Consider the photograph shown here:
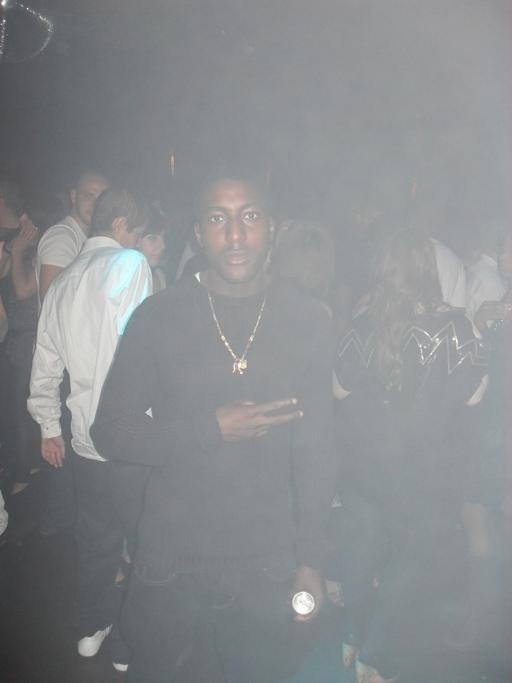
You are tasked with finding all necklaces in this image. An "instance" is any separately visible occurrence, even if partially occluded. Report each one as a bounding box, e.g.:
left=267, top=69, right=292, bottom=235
left=204, top=281, right=266, bottom=373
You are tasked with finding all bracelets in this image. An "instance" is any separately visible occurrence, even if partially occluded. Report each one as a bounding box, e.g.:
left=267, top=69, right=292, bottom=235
left=3, top=242, right=11, bottom=254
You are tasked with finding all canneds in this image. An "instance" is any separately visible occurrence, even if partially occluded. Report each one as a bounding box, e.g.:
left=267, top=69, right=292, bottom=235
left=288, top=590, right=315, bottom=615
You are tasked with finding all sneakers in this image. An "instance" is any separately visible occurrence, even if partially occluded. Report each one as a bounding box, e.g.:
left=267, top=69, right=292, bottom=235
left=75, top=620, right=113, bottom=659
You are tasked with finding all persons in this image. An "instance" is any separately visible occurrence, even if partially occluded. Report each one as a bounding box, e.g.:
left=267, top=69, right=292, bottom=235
left=0, top=164, right=194, bottom=682
left=276, top=165, right=512, bottom=682
left=89, top=167, right=342, bottom=683
